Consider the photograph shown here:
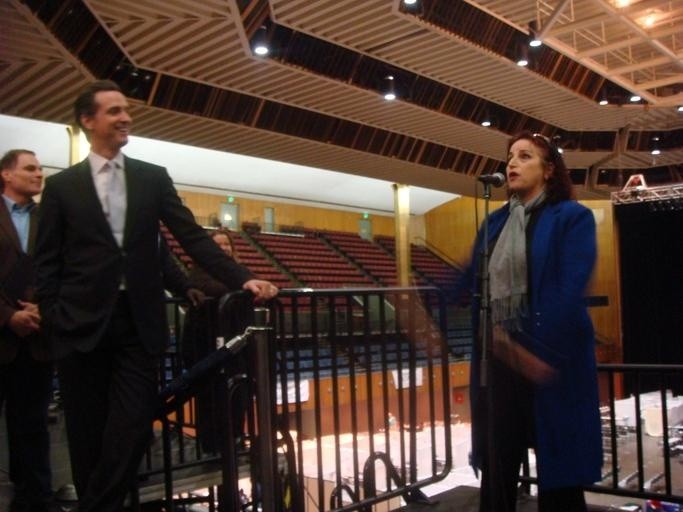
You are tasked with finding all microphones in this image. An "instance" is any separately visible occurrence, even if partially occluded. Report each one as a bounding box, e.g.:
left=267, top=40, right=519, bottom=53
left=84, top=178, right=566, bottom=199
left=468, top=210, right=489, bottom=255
left=478, top=172, right=506, bottom=188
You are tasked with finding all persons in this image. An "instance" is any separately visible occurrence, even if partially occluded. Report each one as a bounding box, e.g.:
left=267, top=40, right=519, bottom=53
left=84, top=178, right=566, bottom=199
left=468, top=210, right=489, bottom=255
left=447, top=131, right=607, bottom=511
left=0, top=149, right=60, bottom=510
left=33, top=78, right=279, bottom=510
left=158, top=243, right=205, bottom=310
left=175, top=229, right=247, bottom=472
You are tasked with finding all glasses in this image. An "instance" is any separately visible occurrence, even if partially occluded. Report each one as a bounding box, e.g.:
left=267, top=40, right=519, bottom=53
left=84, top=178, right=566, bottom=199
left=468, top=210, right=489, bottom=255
left=533, top=133, right=551, bottom=146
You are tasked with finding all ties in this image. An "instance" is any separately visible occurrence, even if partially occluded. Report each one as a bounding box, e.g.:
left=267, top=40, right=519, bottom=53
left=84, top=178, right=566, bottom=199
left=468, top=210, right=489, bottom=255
left=105, top=160, right=128, bottom=249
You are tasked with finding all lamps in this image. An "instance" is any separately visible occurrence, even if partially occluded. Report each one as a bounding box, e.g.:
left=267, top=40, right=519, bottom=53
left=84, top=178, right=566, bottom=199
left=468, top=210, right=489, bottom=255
left=382, top=74, right=396, bottom=102
left=403, top=0, right=681, bottom=158
left=253, top=23, right=271, bottom=57
